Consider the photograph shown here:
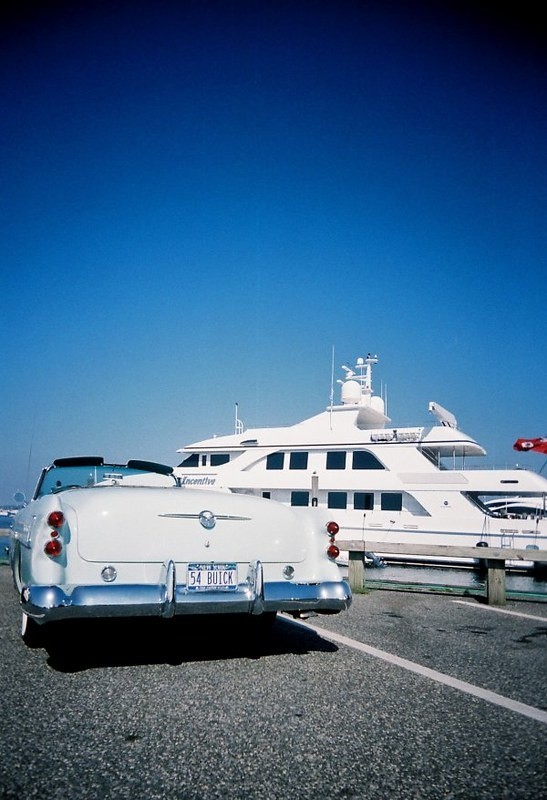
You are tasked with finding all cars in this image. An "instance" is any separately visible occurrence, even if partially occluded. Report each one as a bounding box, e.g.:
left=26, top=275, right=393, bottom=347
left=9, top=454, right=352, bottom=648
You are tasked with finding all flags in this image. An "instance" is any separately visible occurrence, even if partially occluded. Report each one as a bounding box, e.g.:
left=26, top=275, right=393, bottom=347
left=513, top=435, right=547, bottom=454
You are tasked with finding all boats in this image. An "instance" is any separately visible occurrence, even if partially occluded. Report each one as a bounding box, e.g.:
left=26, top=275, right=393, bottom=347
left=169, top=344, right=547, bottom=571
